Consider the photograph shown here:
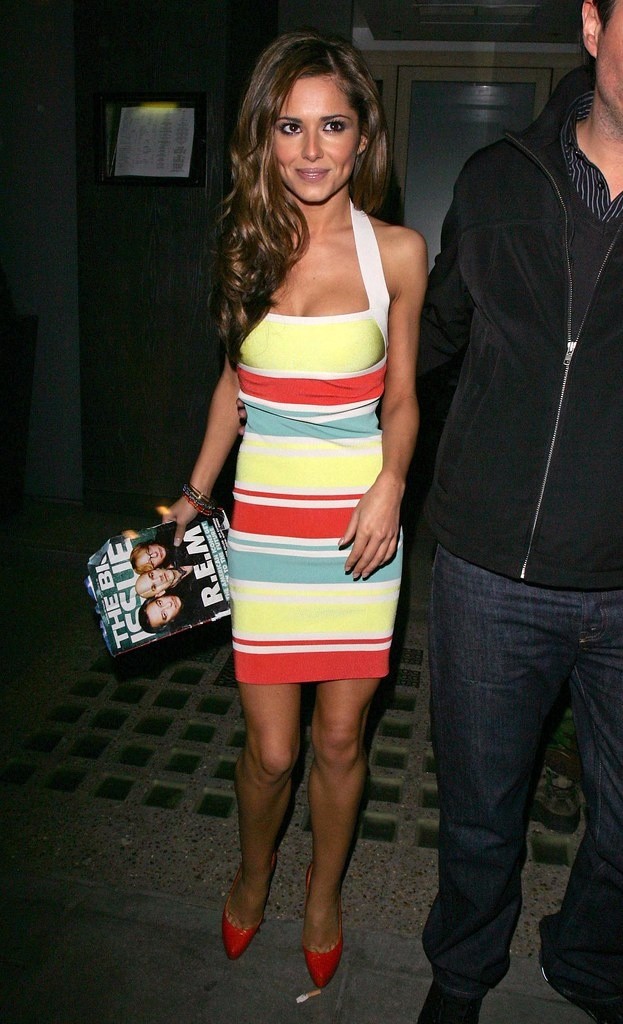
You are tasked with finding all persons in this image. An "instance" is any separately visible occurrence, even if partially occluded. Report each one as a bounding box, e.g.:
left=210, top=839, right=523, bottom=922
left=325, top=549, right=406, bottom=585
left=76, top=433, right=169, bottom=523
left=163, top=29, right=427, bottom=989
left=235, top=0, right=623, bottom=1023
left=130, top=522, right=229, bottom=633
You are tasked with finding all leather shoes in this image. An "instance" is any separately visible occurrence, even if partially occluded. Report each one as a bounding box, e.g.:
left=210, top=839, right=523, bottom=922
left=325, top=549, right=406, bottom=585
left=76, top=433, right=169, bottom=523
left=305, top=864, right=342, bottom=988
left=222, top=852, right=277, bottom=960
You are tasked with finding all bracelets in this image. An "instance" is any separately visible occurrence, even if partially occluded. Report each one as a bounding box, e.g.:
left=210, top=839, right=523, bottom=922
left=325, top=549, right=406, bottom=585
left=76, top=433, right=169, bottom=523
left=181, top=481, right=218, bottom=516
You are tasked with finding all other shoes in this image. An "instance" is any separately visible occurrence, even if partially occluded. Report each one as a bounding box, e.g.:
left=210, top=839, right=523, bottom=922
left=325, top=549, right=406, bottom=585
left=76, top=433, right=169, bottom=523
left=541, top=963, right=623, bottom=1024
left=417, top=980, right=484, bottom=1024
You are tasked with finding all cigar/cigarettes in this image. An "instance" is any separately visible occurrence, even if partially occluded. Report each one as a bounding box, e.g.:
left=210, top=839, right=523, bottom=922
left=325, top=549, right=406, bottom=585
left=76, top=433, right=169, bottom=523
left=296, top=989, right=321, bottom=1003
